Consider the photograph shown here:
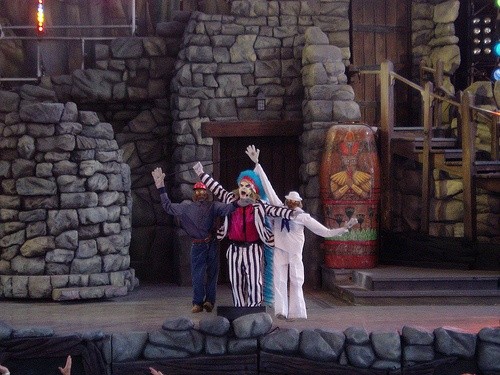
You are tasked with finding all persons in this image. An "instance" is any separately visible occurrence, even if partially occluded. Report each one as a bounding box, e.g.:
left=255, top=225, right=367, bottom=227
left=194, top=161, right=303, bottom=307
left=151, top=167, right=256, bottom=314
left=59, top=355, right=71, bottom=375
left=246, top=145, right=359, bottom=320
left=149, top=367, right=163, bottom=375
left=0, top=365, right=11, bottom=375
